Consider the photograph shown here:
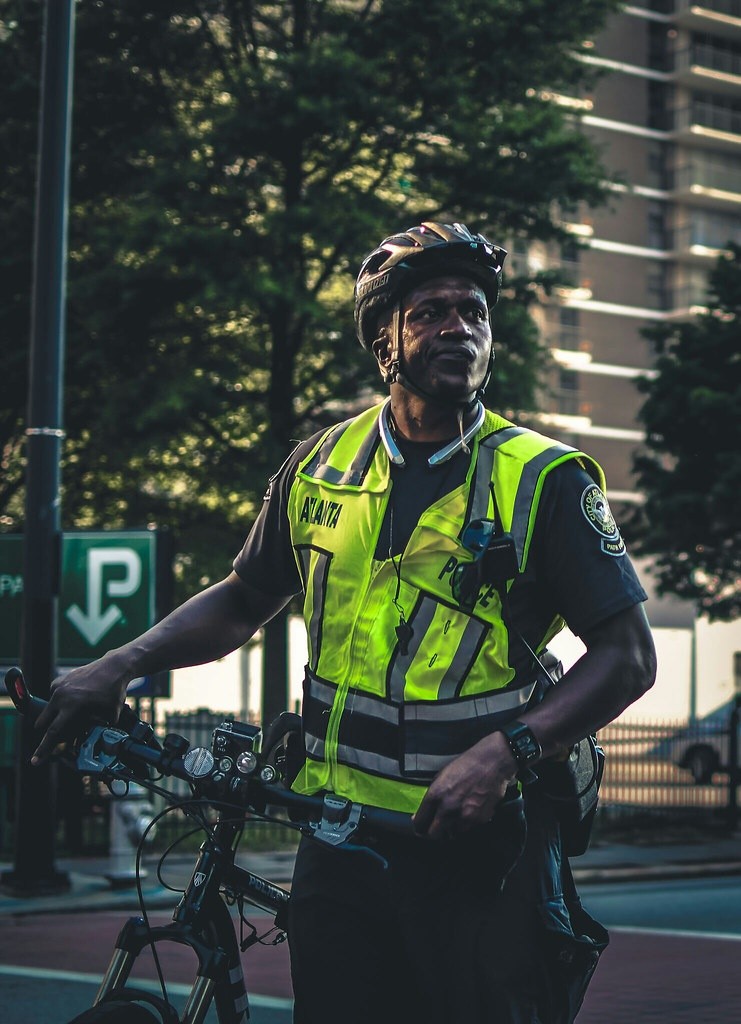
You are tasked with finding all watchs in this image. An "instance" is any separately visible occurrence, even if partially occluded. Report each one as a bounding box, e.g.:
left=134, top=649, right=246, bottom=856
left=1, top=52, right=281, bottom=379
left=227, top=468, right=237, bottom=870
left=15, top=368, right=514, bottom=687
left=500, top=723, right=544, bottom=786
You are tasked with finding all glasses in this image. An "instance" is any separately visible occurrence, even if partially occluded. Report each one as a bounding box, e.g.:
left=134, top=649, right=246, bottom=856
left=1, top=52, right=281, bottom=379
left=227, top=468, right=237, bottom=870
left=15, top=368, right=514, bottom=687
left=451, top=519, right=497, bottom=610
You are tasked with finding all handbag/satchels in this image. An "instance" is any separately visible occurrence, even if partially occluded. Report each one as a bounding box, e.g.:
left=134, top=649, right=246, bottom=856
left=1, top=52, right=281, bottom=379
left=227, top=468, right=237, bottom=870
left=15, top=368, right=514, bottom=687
left=531, top=809, right=610, bottom=1024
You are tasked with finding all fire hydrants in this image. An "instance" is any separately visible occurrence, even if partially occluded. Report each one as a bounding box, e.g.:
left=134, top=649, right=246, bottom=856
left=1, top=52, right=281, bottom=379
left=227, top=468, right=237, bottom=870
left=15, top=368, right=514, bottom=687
left=97, top=775, right=160, bottom=889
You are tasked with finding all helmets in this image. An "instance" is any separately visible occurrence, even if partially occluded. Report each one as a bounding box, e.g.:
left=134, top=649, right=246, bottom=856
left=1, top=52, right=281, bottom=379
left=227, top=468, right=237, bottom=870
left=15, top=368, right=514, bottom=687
left=352, top=221, right=508, bottom=352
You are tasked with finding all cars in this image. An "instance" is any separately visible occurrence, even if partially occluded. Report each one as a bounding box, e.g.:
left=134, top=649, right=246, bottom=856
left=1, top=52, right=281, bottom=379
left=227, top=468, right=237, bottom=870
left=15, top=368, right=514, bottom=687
left=668, top=732, right=732, bottom=784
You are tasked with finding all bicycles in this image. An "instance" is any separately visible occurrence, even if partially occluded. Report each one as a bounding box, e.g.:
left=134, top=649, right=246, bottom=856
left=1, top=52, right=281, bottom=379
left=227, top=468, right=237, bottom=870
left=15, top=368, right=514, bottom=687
left=5, top=664, right=530, bottom=1024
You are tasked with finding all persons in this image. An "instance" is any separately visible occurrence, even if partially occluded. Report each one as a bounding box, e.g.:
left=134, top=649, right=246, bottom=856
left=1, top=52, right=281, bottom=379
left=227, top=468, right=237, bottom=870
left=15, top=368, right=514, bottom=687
left=711, top=651, right=741, bottom=821
left=27, top=218, right=660, bottom=1023
